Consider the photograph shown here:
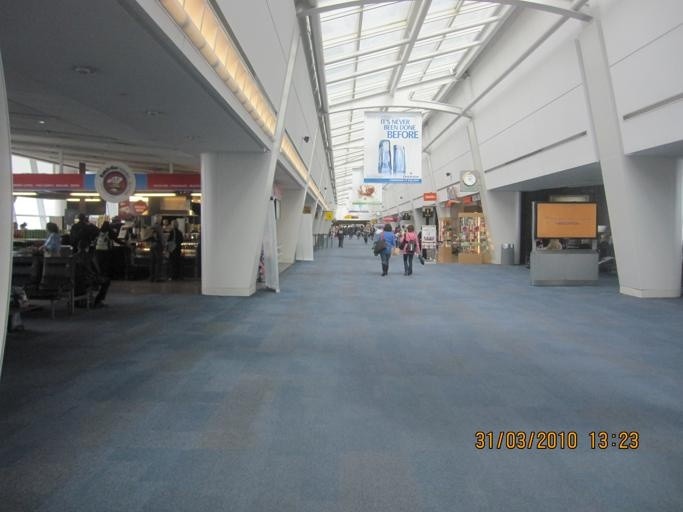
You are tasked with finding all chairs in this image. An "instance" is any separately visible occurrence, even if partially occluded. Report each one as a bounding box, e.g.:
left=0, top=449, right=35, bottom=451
left=9, top=254, right=100, bottom=321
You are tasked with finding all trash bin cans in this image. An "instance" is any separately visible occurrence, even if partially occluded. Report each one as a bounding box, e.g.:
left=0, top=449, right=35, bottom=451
left=501, top=243, right=515, bottom=266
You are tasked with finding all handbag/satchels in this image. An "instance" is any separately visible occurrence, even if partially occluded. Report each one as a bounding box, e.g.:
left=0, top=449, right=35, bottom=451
left=374, top=239, right=386, bottom=256
left=399, top=239, right=406, bottom=250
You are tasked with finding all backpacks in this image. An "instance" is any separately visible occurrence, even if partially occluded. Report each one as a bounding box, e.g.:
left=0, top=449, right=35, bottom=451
left=407, top=240, right=416, bottom=252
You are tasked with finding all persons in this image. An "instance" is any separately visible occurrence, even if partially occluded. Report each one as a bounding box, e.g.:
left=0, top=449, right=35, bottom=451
left=336, top=223, right=427, bottom=276
left=14, top=213, right=184, bottom=309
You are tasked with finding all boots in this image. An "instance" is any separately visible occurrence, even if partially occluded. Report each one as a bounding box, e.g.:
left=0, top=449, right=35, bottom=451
left=381, top=263, right=388, bottom=276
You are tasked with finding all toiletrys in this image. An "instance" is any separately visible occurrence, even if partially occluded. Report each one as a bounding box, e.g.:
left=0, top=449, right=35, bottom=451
left=379, top=139, right=392, bottom=174
left=393, top=144, right=406, bottom=174
